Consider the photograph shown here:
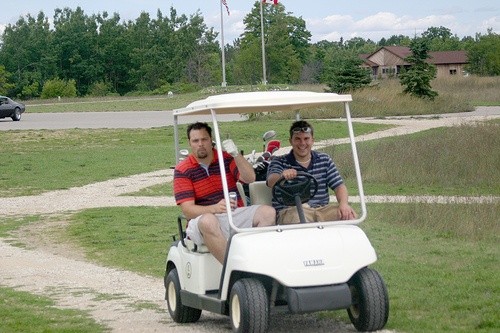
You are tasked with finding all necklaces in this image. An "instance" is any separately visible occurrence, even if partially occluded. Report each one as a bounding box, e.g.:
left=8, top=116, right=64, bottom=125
left=297, top=157, right=312, bottom=164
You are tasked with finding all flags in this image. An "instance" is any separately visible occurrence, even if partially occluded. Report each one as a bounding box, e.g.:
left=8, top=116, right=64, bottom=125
left=222, top=0, right=230, bottom=15
left=262, top=0, right=278, bottom=4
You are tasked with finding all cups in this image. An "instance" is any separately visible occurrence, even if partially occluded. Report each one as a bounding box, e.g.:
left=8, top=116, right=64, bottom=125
left=229, top=192, right=237, bottom=212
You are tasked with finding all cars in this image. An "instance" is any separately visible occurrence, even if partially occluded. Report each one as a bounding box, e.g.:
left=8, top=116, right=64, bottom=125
left=0, top=96, right=26, bottom=121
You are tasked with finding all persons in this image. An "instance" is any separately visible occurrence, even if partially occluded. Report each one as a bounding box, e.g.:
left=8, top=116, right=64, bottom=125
left=267, top=120, right=356, bottom=225
left=173, top=123, right=276, bottom=267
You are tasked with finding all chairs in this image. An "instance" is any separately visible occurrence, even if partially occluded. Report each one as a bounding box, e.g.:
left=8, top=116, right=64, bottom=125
left=249, top=181, right=274, bottom=207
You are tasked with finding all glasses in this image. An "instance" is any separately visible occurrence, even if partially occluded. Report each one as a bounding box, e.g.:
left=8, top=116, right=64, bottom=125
left=291, top=127, right=313, bottom=133
